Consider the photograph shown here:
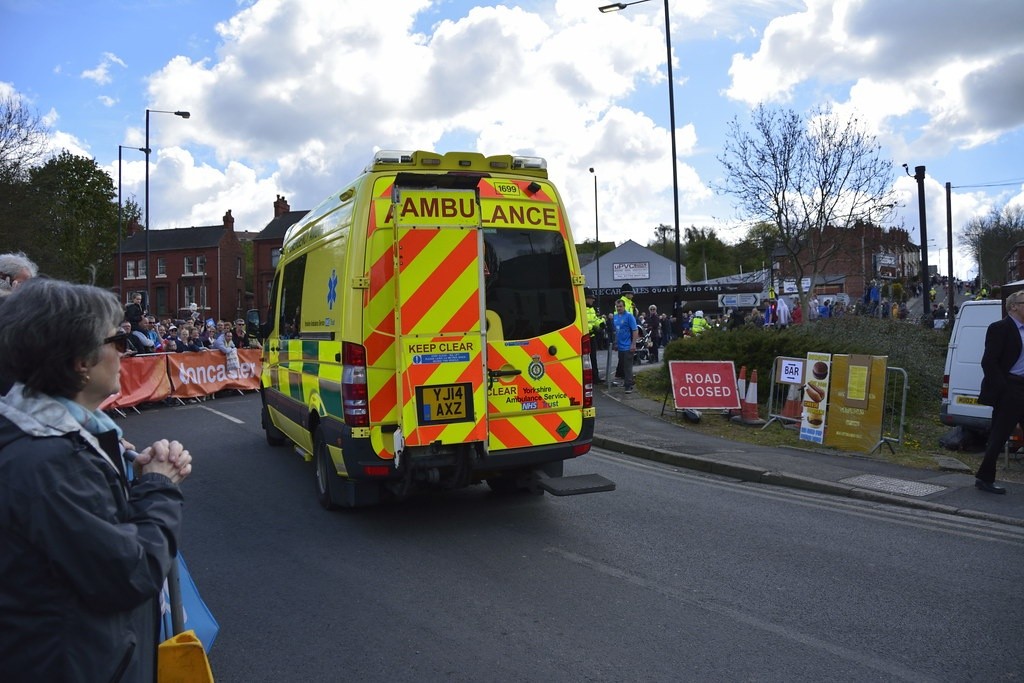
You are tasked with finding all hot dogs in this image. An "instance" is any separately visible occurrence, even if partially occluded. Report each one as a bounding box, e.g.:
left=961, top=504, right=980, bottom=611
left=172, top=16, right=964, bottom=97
left=804, top=382, right=825, bottom=402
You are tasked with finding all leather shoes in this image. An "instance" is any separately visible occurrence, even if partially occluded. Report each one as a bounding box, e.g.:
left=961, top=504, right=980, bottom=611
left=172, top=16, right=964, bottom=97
left=975, top=478, right=1006, bottom=494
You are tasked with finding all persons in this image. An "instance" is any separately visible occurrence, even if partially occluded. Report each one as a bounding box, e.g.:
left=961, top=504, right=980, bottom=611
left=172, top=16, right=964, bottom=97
left=0, top=251, right=266, bottom=683
left=579, top=271, right=1023, bottom=493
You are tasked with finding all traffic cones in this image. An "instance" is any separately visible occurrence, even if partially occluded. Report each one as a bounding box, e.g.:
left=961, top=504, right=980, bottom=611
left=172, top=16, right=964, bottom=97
left=725, top=366, right=748, bottom=419
left=738, top=368, right=766, bottom=426
left=780, top=380, right=804, bottom=432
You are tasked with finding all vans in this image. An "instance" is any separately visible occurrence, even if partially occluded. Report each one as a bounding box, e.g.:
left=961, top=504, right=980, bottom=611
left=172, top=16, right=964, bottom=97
left=939, top=299, right=1024, bottom=453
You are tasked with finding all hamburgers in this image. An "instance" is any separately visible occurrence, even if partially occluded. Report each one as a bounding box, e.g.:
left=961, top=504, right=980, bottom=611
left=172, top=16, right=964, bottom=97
left=813, top=362, right=828, bottom=380
left=807, top=411, right=823, bottom=427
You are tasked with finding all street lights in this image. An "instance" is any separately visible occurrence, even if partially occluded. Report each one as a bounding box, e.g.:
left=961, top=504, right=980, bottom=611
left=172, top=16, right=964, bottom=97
left=115, top=143, right=153, bottom=300
left=599, top=0, right=690, bottom=340
left=589, top=167, right=601, bottom=314
left=145, top=106, right=193, bottom=333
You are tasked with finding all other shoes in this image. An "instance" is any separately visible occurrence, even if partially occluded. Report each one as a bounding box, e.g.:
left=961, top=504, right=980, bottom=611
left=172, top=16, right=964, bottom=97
left=647, top=359, right=659, bottom=364
left=593, top=378, right=637, bottom=394
left=147, top=394, right=211, bottom=406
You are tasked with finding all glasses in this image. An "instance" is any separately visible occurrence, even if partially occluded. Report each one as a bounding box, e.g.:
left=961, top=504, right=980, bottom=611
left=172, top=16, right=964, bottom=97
left=149, top=322, right=155, bottom=324
left=650, top=308, right=655, bottom=310
left=104, top=331, right=130, bottom=353
left=224, top=328, right=229, bottom=329
left=209, top=331, right=214, bottom=333
left=238, top=324, right=244, bottom=326
left=187, top=322, right=194, bottom=324
left=1015, top=301, right=1024, bottom=304
left=169, top=329, right=177, bottom=332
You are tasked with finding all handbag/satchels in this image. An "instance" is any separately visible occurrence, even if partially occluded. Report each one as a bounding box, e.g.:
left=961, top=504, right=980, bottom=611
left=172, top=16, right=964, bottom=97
left=126, top=454, right=221, bottom=657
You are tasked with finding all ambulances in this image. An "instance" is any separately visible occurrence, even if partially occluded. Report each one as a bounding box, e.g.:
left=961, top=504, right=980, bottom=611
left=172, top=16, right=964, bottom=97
left=246, top=153, right=616, bottom=513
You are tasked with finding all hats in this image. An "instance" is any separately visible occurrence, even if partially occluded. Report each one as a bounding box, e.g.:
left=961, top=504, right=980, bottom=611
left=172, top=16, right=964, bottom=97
left=169, top=325, right=177, bottom=329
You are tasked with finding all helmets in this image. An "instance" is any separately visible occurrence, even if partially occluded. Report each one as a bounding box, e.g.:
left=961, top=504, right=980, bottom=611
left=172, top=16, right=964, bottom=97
left=620, top=284, right=634, bottom=293
left=695, top=310, right=703, bottom=317
left=584, top=287, right=597, bottom=299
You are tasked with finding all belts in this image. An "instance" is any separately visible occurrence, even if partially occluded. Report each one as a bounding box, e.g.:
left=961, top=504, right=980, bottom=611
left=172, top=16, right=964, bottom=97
left=1008, top=375, right=1024, bottom=383
